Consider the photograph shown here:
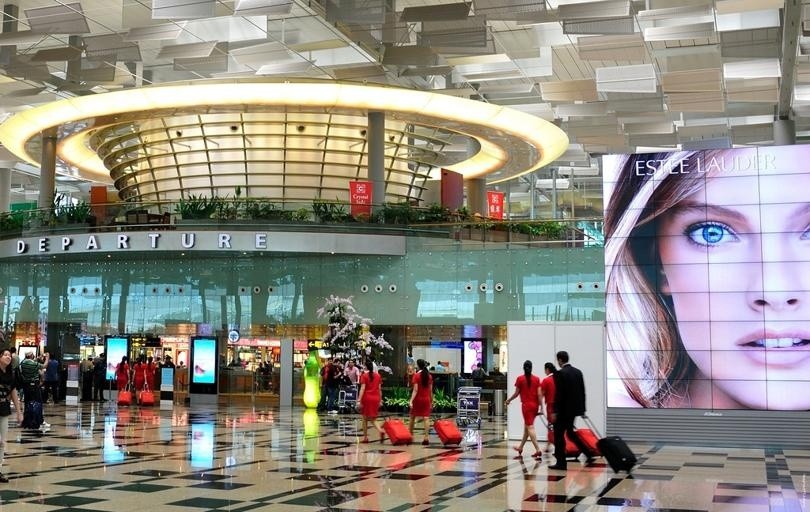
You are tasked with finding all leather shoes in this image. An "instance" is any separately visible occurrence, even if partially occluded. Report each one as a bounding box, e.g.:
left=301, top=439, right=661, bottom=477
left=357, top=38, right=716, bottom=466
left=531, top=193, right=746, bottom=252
left=547, top=462, right=570, bottom=470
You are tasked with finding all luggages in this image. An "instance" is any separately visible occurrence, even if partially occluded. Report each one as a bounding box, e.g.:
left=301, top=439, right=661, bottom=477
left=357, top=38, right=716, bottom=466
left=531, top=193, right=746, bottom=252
left=384, top=418, right=413, bottom=446
left=433, top=418, right=463, bottom=446
left=548, top=428, right=601, bottom=458
left=117, top=382, right=133, bottom=405
left=597, top=435, right=637, bottom=475
left=25, top=400, right=42, bottom=429
left=140, top=384, right=155, bottom=406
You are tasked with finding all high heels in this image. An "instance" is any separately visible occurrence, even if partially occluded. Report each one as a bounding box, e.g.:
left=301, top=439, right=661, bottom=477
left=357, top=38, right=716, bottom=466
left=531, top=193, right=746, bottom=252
left=357, top=429, right=430, bottom=446
left=512, top=441, right=553, bottom=458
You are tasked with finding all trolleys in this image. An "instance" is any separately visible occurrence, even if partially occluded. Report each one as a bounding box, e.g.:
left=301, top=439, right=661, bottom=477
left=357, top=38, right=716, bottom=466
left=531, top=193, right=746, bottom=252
left=453, top=386, right=483, bottom=431
left=339, top=384, right=360, bottom=414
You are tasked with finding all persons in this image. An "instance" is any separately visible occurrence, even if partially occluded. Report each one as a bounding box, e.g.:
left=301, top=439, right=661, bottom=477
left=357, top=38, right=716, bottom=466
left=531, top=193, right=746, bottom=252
left=489, top=366, right=504, bottom=376
left=0, top=348, right=24, bottom=483
left=356, top=360, right=385, bottom=444
left=426, top=361, right=445, bottom=372
left=408, top=358, right=434, bottom=445
left=10, top=346, right=175, bottom=405
left=20, top=351, right=51, bottom=429
left=318, top=357, right=360, bottom=414
left=604, top=144, right=810, bottom=410
left=471, top=363, right=487, bottom=387
left=228, top=357, right=272, bottom=393
left=541, top=362, right=558, bottom=453
left=548, top=350, right=596, bottom=470
left=505, top=360, right=542, bottom=458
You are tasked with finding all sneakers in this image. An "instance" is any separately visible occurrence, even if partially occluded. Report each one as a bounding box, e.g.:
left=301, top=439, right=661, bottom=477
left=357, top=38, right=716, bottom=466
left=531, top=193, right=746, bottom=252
left=0, top=472, right=9, bottom=483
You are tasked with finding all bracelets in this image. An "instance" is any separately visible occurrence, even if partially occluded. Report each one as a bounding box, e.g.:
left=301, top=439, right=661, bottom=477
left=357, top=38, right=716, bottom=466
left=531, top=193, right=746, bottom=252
left=357, top=401, right=360, bottom=402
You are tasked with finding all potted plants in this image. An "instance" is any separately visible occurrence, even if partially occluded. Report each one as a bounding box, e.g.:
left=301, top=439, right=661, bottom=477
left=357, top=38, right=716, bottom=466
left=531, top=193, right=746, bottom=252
left=381, top=385, right=459, bottom=413
